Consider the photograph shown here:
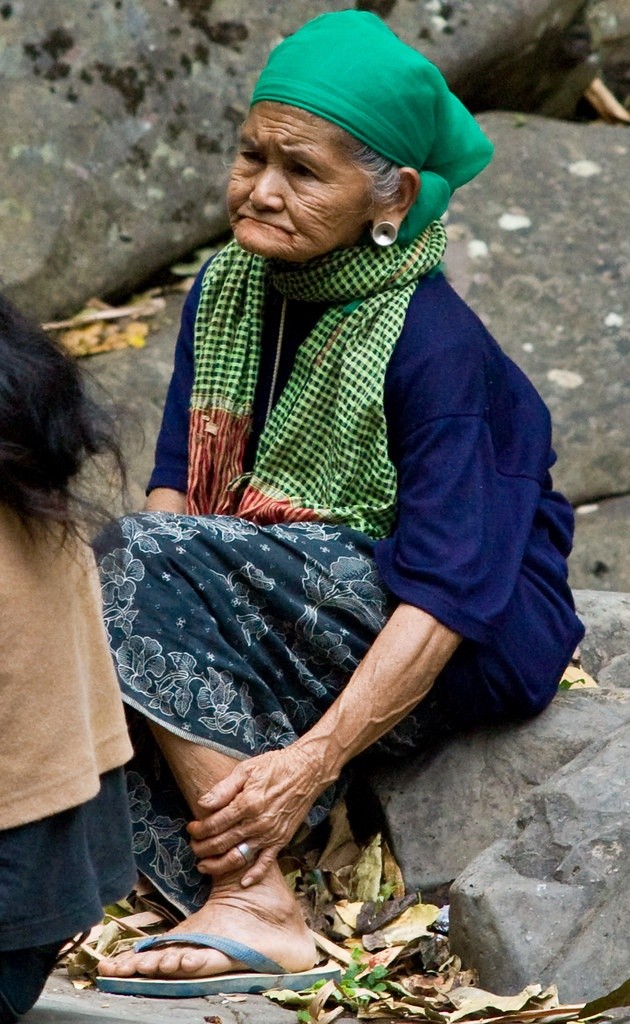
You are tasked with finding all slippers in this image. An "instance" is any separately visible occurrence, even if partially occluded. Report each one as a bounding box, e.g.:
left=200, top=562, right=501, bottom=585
left=94, top=933, right=340, bottom=997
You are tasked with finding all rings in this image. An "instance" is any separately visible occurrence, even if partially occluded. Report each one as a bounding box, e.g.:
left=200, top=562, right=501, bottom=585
left=237, top=842, right=254, bottom=863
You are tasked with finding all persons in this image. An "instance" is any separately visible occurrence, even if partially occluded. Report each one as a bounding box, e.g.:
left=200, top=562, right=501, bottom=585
left=0, top=297, right=139, bottom=1024
left=66, top=9, right=587, bottom=997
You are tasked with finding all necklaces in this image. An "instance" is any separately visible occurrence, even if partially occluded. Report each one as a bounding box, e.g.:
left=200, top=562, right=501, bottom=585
left=264, top=296, right=287, bottom=424
